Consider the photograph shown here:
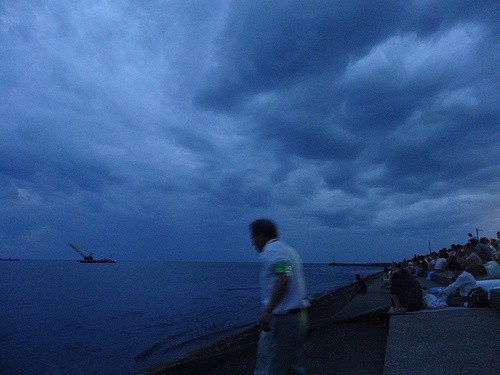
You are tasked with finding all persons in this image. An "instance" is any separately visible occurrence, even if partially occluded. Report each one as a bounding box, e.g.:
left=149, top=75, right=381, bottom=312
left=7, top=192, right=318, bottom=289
left=349, top=274, right=367, bottom=300
left=250, top=219, right=309, bottom=375
left=381, top=231, right=500, bottom=313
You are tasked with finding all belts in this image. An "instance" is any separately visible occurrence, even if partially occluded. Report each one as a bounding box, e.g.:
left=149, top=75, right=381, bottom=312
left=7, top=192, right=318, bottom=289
left=288, top=309, right=302, bottom=315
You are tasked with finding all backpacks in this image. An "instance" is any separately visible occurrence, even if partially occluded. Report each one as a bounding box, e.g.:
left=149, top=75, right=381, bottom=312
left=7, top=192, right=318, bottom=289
left=466, top=287, right=487, bottom=308
